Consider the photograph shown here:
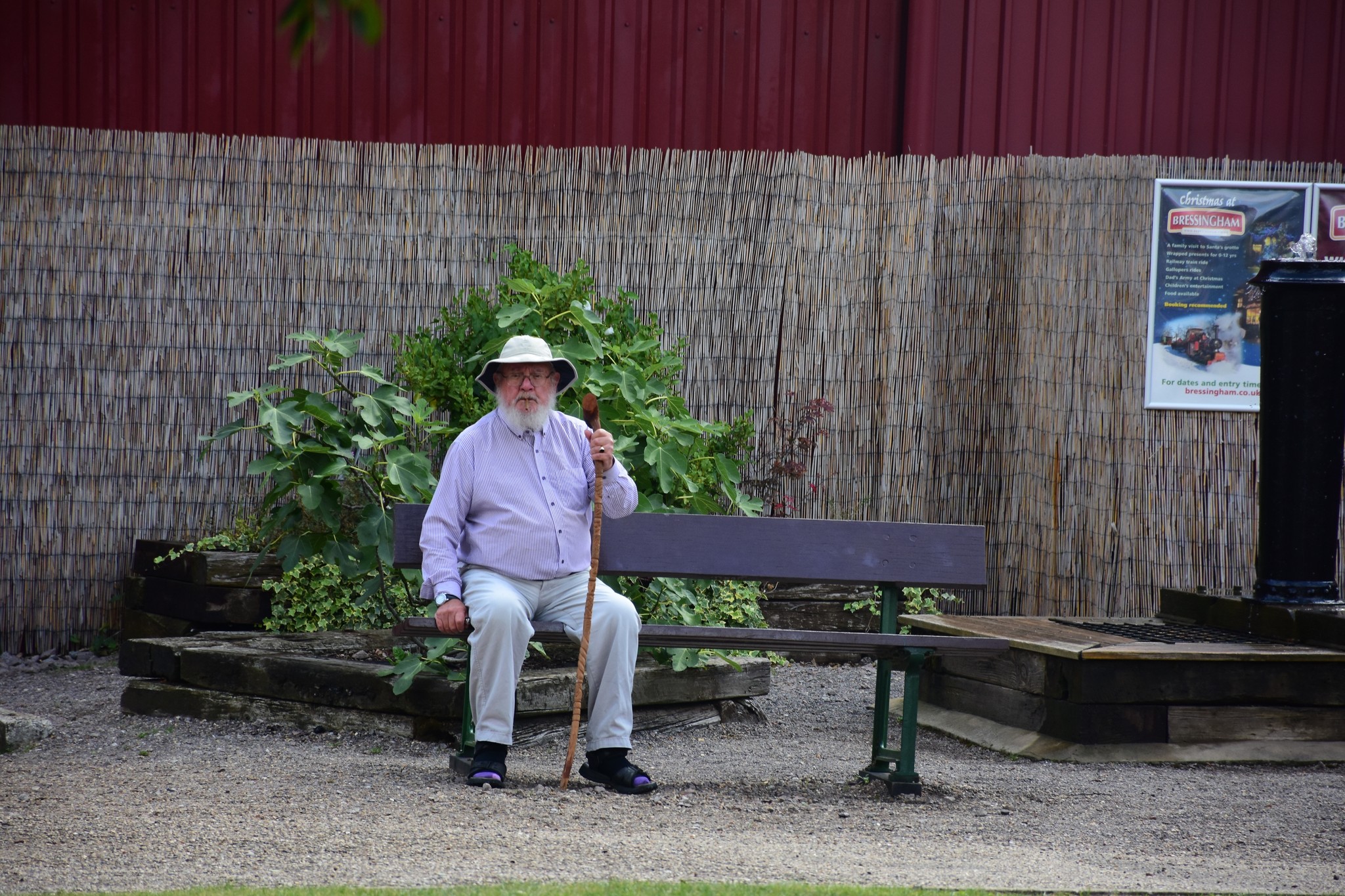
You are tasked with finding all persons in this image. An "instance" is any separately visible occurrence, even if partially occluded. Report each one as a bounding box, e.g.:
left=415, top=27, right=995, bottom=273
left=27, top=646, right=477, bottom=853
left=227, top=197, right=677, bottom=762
left=419, top=335, right=659, bottom=795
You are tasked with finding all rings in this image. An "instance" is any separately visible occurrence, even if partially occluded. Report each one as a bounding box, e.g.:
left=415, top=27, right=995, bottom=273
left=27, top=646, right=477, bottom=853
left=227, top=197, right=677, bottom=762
left=599, top=446, right=605, bottom=453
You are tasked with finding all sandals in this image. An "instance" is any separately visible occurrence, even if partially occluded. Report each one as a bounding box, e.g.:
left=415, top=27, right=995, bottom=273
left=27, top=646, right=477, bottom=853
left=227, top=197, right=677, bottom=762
left=578, top=747, right=658, bottom=793
left=465, top=740, right=508, bottom=788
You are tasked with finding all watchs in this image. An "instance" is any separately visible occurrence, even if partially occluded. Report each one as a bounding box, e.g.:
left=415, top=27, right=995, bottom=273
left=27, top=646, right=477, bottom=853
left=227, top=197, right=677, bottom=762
left=435, top=592, right=460, bottom=608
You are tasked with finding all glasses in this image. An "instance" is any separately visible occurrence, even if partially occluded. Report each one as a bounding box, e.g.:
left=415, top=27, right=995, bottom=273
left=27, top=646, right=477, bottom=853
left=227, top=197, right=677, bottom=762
left=497, top=369, right=553, bottom=387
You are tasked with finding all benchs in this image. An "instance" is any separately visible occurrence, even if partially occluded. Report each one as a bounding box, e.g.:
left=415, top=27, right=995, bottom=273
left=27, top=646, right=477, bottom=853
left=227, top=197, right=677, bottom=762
left=393, top=501, right=1010, bottom=798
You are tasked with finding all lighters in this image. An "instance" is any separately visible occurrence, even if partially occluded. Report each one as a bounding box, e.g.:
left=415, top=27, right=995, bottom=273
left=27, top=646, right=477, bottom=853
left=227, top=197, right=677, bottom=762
left=464, top=617, right=470, bottom=625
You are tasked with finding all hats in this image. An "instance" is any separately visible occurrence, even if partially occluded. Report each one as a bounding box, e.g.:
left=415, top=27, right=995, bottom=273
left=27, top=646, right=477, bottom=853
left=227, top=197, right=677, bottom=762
left=475, top=334, right=578, bottom=398
